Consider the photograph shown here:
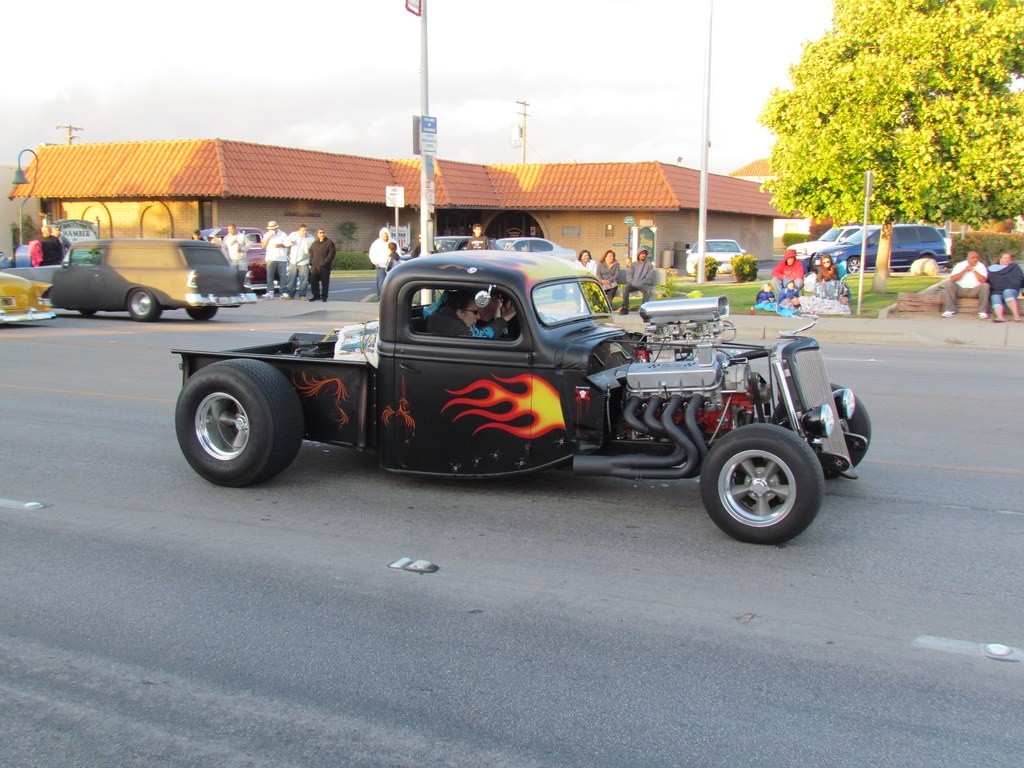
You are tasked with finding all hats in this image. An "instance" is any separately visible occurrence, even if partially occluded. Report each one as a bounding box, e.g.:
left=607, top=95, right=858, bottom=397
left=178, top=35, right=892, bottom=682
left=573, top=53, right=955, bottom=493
left=474, top=287, right=502, bottom=312
left=267, top=221, right=279, bottom=229
left=194, top=229, right=200, bottom=235
left=441, top=290, right=475, bottom=310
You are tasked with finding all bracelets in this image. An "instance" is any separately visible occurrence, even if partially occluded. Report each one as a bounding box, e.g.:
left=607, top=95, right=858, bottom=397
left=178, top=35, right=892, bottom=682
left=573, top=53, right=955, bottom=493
left=972, top=269, right=976, bottom=273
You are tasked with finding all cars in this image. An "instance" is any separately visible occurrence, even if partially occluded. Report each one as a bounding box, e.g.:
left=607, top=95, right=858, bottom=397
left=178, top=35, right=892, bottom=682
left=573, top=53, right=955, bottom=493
left=812, top=224, right=949, bottom=278
left=3, top=238, right=262, bottom=319
left=787, top=226, right=862, bottom=274
left=390, top=237, right=502, bottom=280
left=496, top=237, right=578, bottom=268
left=196, top=227, right=311, bottom=293
left=685, top=239, right=747, bottom=278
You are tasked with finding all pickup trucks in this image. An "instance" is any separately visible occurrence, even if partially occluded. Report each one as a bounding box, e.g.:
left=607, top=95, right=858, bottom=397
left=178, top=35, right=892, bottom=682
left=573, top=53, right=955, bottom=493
left=169, top=251, right=873, bottom=547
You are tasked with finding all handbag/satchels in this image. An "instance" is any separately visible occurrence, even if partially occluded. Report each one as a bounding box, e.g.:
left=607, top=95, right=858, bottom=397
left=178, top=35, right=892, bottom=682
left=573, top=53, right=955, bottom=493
left=603, top=281, right=618, bottom=291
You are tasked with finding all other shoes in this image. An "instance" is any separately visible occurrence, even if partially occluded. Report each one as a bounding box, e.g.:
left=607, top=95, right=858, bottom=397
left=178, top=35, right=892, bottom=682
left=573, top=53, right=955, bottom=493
left=619, top=308, right=628, bottom=315
left=1015, top=318, right=1021, bottom=321
left=323, top=297, right=326, bottom=302
left=283, top=295, right=293, bottom=300
left=976, top=312, right=989, bottom=319
left=280, top=292, right=289, bottom=298
left=299, top=295, right=306, bottom=301
left=993, top=317, right=1006, bottom=321
left=309, top=296, right=320, bottom=301
left=261, top=291, right=274, bottom=298
left=941, top=311, right=955, bottom=318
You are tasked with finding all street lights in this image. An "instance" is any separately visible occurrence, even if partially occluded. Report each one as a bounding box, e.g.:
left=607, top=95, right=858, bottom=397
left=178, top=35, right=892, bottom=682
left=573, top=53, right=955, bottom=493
left=12, top=146, right=38, bottom=247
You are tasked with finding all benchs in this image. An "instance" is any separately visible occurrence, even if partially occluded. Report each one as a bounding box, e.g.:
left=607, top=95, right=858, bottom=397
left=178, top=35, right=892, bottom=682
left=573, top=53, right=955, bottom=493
left=410, top=306, right=427, bottom=334
left=897, top=292, right=1024, bottom=316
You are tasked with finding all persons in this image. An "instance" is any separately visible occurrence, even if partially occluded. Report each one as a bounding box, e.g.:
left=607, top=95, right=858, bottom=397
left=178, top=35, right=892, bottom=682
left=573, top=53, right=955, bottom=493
left=426, top=289, right=517, bottom=340
left=368, top=227, right=397, bottom=301
left=411, top=234, right=438, bottom=259
left=575, top=249, right=597, bottom=276
left=618, top=247, right=655, bottom=315
left=987, top=251, right=1024, bottom=322
left=595, top=250, right=620, bottom=307
left=465, top=224, right=491, bottom=250
left=384, top=242, right=401, bottom=276
left=192, top=219, right=336, bottom=302
left=754, top=249, right=840, bottom=310
left=31, top=223, right=71, bottom=267
left=940, top=250, right=990, bottom=318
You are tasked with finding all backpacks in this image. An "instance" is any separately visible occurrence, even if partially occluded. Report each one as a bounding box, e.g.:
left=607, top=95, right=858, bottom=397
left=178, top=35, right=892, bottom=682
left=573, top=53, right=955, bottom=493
left=387, top=255, right=402, bottom=267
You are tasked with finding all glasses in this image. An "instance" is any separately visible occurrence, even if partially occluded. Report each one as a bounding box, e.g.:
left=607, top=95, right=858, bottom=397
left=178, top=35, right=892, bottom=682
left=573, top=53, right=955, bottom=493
left=465, top=310, right=480, bottom=315
left=317, top=231, right=325, bottom=234
left=823, top=261, right=829, bottom=264
